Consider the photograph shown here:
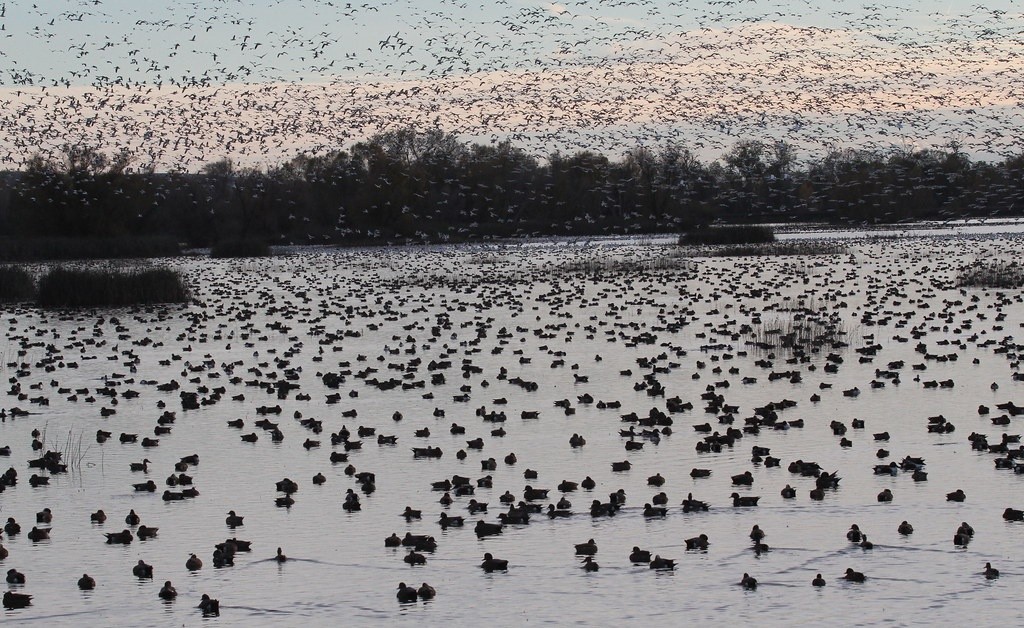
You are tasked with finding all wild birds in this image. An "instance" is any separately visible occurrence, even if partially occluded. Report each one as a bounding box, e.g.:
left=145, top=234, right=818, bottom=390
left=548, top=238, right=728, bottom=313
left=0, top=2, right=1024, bottom=296
left=0, top=295, right=1024, bottom=627
